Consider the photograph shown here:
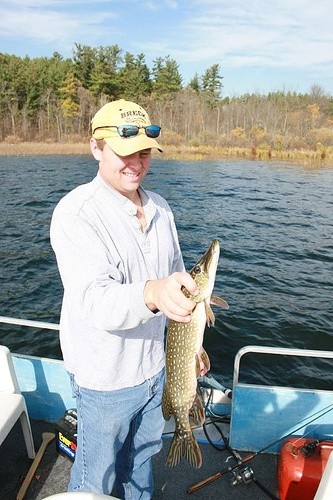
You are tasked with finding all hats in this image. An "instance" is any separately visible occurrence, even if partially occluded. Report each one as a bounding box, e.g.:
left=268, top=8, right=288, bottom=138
left=92, top=98, right=163, bottom=157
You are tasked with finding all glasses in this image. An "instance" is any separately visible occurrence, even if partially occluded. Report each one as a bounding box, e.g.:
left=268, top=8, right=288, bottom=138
left=93, top=125, right=161, bottom=138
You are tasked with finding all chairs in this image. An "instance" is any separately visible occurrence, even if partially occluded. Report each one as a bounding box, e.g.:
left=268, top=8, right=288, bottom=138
left=0, top=344, right=36, bottom=460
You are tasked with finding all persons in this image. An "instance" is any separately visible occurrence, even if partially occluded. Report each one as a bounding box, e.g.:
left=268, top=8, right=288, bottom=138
left=49, top=99, right=199, bottom=500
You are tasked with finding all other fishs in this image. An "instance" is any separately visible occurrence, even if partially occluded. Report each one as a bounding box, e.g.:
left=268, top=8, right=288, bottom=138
left=161, top=238, right=230, bottom=471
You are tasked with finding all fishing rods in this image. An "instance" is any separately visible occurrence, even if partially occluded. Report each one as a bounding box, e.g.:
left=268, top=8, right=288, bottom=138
left=189, top=403, right=333, bottom=492
